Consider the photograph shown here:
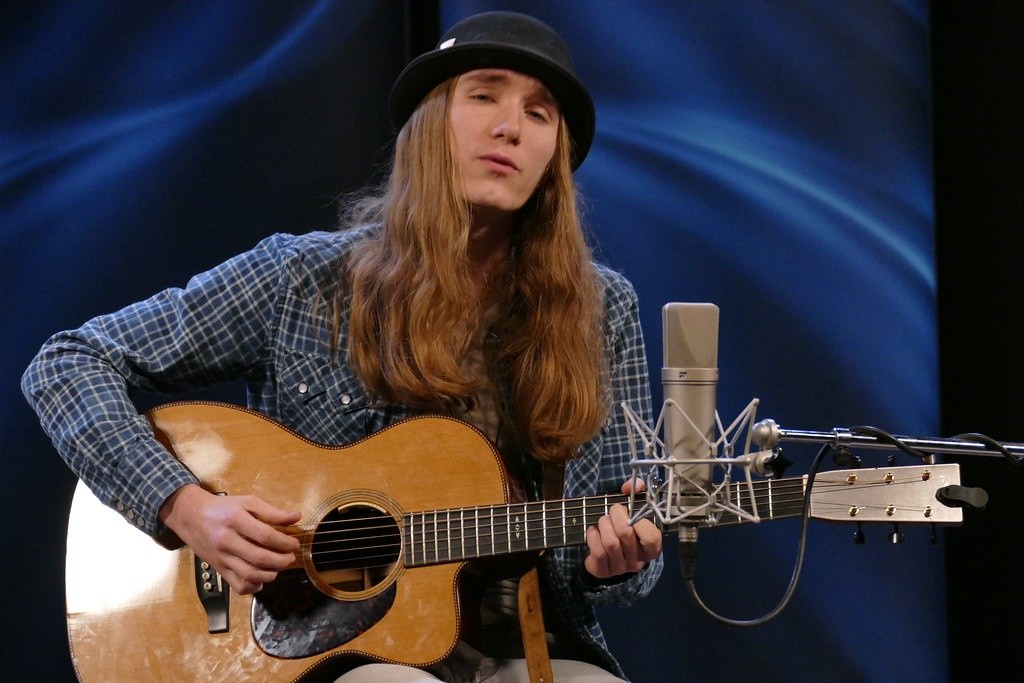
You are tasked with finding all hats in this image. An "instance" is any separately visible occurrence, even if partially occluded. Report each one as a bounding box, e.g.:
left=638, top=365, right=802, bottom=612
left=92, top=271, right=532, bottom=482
left=389, top=10, right=596, bottom=175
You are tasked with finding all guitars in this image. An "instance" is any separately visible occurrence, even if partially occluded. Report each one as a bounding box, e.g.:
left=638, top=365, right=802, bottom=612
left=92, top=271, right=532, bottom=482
left=64, top=400, right=989, bottom=682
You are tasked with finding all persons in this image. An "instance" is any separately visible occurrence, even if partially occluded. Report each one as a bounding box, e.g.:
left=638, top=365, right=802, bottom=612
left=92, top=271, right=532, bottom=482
left=20, top=11, right=665, bottom=683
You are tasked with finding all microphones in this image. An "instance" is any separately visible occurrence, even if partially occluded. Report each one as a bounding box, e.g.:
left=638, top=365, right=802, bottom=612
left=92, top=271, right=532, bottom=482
left=661, top=303, right=719, bottom=518
